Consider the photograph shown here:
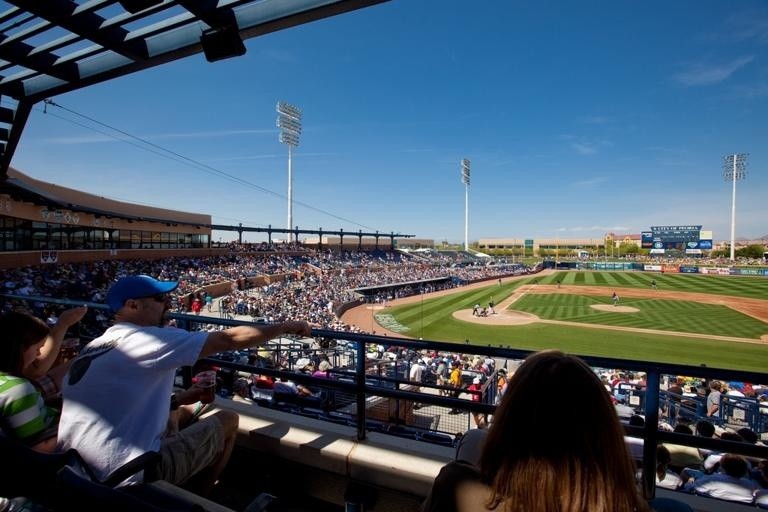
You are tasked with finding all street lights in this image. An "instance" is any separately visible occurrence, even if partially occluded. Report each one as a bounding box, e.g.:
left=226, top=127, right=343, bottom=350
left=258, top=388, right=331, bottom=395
left=460, top=157, right=472, bottom=251
left=274, top=99, right=304, bottom=245
left=720, top=151, right=750, bottom=261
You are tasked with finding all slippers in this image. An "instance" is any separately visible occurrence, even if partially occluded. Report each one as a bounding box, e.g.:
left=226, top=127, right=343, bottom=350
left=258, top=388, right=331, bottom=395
left=187, top=401, right=211, bottom=423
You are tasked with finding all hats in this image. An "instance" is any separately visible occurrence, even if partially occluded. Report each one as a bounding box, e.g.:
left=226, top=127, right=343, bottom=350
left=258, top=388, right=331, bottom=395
left=294, top=358, right=310, bottom=370
left=234, top=377, right=252, bottom=390
left=106, top=274, right=179, bottom=314
left=318, top=361, right=329, bottom=371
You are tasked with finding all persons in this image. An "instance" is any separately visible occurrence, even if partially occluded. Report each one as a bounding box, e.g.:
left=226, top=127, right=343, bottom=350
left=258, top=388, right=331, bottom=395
left=533, top=254, right=767, bottom=269
left=612, top=292, right=619, bottom=305
left=556, top=279, right=560, bottom=289
left=471, top=301, right=496, bottom=318
left=421, top=347, right=649, bottom=510
left=533, top=277, right=539, bottom=285
left=651, top=279, right=657, bottom=289
left=346, top=322, right=508, bottom=429
left=346, top=244, right=532, bottom=308
left=1, top=240, right=346, bottom=509
left=660, top=269, right=665, bottom=275
left=597, top=367, right=768, bottom=504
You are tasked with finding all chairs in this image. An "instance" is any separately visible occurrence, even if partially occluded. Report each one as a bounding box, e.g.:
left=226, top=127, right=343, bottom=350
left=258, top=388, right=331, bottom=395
left=86, top=452, right=366, bottom=512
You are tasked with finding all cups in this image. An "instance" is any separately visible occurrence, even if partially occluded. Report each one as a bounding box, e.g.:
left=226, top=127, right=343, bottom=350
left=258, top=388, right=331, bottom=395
left=194, top=370, right=216, bottom=404
